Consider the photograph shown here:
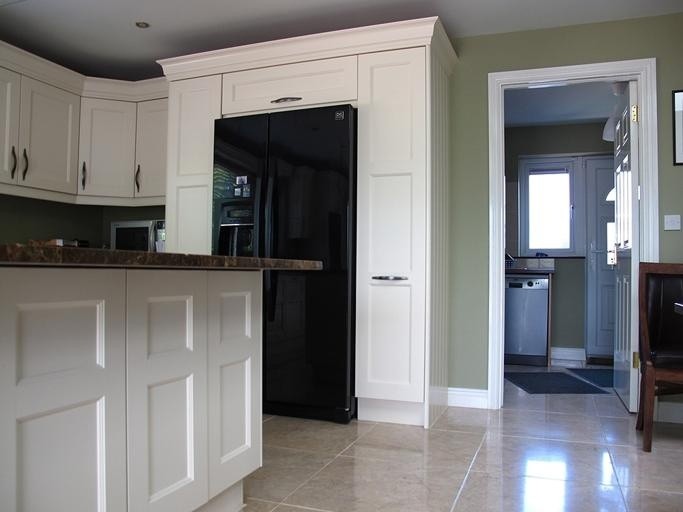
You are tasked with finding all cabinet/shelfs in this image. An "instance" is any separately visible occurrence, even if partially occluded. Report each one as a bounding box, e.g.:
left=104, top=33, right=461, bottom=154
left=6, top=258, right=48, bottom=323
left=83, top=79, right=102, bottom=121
left=222, top=26, right=358, bottom=118
left=154, top=48, right=221, bottom=255
left=79, top=73, right=134, bottom=207
left=0, top=38, right=83, bottom=203
left=134, top=75, right=167, bottom=207
left=347, top=14, right=463, bottom=427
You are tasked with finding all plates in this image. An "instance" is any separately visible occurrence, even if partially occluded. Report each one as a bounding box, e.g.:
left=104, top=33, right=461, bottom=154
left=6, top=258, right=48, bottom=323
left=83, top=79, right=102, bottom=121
left=28, top=238, right=88, bottom=247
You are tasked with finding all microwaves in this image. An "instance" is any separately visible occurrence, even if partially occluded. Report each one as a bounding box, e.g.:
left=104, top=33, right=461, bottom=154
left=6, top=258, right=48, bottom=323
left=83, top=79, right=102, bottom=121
left=110, top=216, right=165, bottom=252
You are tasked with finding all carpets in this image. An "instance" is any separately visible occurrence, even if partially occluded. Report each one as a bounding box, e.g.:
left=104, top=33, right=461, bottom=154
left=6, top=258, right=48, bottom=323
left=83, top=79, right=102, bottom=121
left=504, top=371, right=613, bottom=398
left=566, top=364, right=613, bottom=388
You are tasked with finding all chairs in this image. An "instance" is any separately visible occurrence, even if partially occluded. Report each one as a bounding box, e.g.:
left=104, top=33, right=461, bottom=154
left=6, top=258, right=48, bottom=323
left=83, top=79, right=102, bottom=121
left=635, top=262, right=682, bottom=453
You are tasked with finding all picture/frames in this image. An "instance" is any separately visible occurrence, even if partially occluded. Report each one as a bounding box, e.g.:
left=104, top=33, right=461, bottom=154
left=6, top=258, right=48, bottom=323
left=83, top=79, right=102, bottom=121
left=672, top=90, right=682, bottom=166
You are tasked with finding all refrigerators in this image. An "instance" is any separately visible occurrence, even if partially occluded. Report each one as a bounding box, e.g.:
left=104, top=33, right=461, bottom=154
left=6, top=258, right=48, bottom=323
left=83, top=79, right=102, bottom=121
left=210, top=106, right=355, bottom=422
